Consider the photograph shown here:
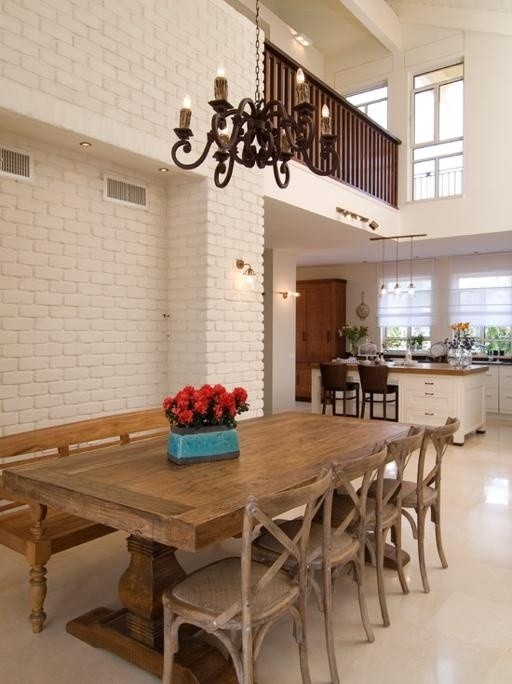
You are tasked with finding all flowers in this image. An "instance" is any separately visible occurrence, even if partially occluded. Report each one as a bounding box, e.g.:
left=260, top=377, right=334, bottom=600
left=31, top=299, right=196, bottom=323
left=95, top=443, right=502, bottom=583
left=163, top=383, right=249, bottom=429
left=448, top=322, right=470, bottom=336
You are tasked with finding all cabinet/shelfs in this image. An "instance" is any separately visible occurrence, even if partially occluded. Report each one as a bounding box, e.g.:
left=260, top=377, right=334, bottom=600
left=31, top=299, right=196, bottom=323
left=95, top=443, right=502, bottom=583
left=399, top=372, right=486, bottom=445
left=305, top=282, right=333, bottom=358
left=296, top=280, right=305, bottom=358
left=499, top=365, right=512, bottom=415
left=485, top=363, right=499, bottom=414
left=296, top=361, right=311, bottom=400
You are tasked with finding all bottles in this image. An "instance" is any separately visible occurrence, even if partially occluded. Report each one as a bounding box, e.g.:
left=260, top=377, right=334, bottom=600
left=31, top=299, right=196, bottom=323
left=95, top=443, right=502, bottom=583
left=375, top=352, right=379, bottom=364
left=379, top=353, right=384, bottom=365
left=406, top=345, right=411, bottom=360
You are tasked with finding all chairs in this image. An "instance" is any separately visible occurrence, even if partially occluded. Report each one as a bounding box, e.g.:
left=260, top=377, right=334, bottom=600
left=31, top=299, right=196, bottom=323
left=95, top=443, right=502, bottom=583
left=317, top=425, right=426, bottom=627
left=159, top=467, right=335, bottom=684
left=319, top=363, right=360, bottom=419
left=247, top=440, right=387, bottom=683
left=356, top=363, right=401, bottom=422
left=359, top=416, right=458, bottom=582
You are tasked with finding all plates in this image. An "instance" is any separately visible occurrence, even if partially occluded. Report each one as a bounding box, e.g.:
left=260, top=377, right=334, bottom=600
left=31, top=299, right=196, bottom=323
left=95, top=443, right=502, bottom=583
left=430, top=341, right=447, bottom=357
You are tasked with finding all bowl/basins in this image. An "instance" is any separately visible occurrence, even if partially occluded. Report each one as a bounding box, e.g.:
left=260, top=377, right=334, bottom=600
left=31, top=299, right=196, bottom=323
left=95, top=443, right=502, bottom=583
left=408, top=361, right=417, bottom=366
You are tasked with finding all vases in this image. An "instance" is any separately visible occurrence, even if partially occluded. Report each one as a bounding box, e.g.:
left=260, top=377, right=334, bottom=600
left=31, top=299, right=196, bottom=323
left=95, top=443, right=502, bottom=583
left=167, top=427, right=241, bottom=464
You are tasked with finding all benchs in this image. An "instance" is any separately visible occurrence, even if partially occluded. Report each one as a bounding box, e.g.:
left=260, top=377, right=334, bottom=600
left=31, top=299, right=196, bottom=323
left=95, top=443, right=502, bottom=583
left=0, top=406, right=173, bottom=633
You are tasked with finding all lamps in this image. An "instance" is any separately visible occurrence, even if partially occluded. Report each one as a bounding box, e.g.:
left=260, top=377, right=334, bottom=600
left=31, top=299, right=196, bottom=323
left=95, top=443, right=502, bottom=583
left=380, top=238, right=387, bottom=298
left=409, top=237, right=414, bottom=295
left=275, top=291, right=289, bottom=299
left=236, top=259, right=257, bottom=285
left=393, top=237, right=401, bottom=294
left=171, top=3, right=342, bottom=189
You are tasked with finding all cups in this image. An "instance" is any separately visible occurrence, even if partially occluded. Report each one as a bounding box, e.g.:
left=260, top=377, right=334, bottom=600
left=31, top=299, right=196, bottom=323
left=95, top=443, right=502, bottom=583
left=487, top=347, right=504, bottom=361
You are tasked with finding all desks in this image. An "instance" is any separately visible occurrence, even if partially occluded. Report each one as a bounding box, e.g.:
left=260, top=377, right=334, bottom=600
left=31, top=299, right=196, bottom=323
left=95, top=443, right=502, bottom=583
left=0, top=412, right=417, bottom=684
